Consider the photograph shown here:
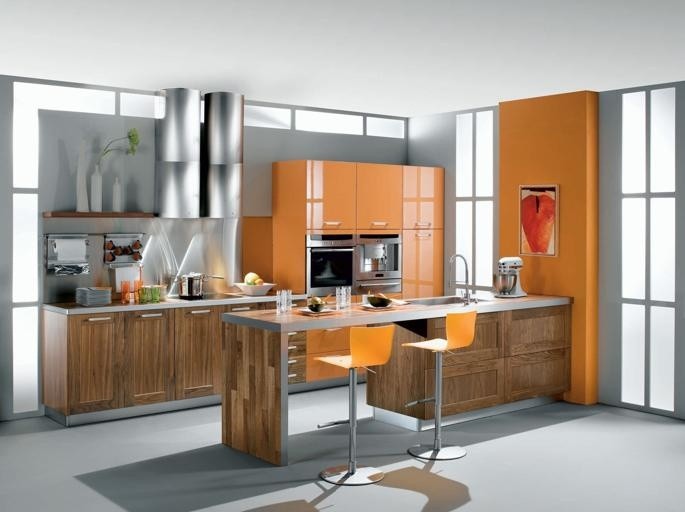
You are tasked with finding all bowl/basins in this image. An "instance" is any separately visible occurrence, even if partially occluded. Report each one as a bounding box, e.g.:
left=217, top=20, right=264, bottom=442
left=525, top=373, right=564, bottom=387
left=367, top=297, right=392, bottom=308
left=232, top=283, right=276, bottom=296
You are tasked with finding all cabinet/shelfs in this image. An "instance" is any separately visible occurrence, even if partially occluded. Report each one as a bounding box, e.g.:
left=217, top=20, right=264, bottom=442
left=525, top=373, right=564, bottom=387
left=124, top=309, right=175, bottom=407
left=356, top=162, right=402, bottom=230
left=67, top=312, right=125, bottom=414
left=306, top=160, right=356, bottom=229
left=403, top=229, right=443, bottom=299
left=307, top=299, right=366, bottom=381
left=266, top=300, right=305, bottom=385
left=403, top=166, right=444, bottom=228
left=173, top=307, right=219, bottom=400
left=218, top=304, right=264, bottom=395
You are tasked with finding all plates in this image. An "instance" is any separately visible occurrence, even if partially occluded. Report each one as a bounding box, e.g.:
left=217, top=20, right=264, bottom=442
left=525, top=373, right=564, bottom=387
left=75, top=288, right=112, bottom=305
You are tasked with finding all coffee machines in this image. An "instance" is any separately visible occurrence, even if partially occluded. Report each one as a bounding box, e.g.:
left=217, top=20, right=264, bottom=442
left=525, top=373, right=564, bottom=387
left=495, top=256, right=528, bottom=298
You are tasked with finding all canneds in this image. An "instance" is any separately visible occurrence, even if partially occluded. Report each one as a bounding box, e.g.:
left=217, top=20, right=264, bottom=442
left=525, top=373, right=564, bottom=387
left=307, top=296, right=313, bottom=309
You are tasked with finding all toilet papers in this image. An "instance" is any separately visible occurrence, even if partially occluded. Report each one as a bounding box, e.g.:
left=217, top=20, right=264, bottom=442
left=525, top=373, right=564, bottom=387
left=52, top=239, right=87, bottom=261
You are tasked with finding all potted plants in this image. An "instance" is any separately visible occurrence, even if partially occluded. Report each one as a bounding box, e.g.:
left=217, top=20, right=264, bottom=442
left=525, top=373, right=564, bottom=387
left=90, top=127, right=138, bottom=213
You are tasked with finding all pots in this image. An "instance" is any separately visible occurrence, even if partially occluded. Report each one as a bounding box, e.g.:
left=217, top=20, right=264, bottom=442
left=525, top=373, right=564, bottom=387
left=172, top=272, right=224, bottom=298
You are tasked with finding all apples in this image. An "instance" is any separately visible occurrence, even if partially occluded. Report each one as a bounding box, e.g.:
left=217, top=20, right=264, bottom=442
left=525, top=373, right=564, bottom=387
left=311, top=296, right=321, bottom=304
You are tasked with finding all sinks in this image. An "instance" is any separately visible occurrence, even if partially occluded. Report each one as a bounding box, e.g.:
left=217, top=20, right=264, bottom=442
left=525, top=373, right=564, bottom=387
left=404, top=297, right=485, bottom=306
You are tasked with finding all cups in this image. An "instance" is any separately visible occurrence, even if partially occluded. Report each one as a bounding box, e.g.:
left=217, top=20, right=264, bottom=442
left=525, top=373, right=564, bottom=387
left=133, top=279, right=166, bottom=304
left=276, top=289, right=292, bottom=312
left=308, top=303, right=326, bottom=313
left=120, top=280, right=130, bottom=303
left=335, top=286, right=351, bottom=309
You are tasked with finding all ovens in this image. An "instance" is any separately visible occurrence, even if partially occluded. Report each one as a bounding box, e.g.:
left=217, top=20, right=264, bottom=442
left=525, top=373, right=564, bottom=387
left=307, top=235, right=402, bottom=295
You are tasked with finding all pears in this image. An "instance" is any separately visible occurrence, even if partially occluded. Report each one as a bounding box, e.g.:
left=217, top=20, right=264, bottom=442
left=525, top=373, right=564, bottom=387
left=255, top=279, right=263, bottom=285
left=376, top=294, right=387, bottom=300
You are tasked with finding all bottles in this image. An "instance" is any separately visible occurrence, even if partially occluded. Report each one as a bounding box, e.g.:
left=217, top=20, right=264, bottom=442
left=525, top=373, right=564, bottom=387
left=111, top=176, right=122, bottom=214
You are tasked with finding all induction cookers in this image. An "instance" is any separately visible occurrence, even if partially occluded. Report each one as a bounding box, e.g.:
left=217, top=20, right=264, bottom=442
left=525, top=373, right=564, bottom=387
left=168, top=292, right=242, bottom=302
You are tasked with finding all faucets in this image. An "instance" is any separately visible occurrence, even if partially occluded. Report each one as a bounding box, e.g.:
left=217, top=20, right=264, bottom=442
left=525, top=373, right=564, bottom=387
left=450, top=254, right=468, bottom=300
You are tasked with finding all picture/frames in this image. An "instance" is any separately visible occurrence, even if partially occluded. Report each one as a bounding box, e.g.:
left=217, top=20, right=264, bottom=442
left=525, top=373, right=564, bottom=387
left=516, top=184, right=559, bottom=256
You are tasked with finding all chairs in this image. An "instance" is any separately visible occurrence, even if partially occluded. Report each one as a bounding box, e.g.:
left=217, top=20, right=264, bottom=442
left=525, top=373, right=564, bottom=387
left=313, top=325, right=394, bottom=485
left=401, top=310, right=476, bottom=461
